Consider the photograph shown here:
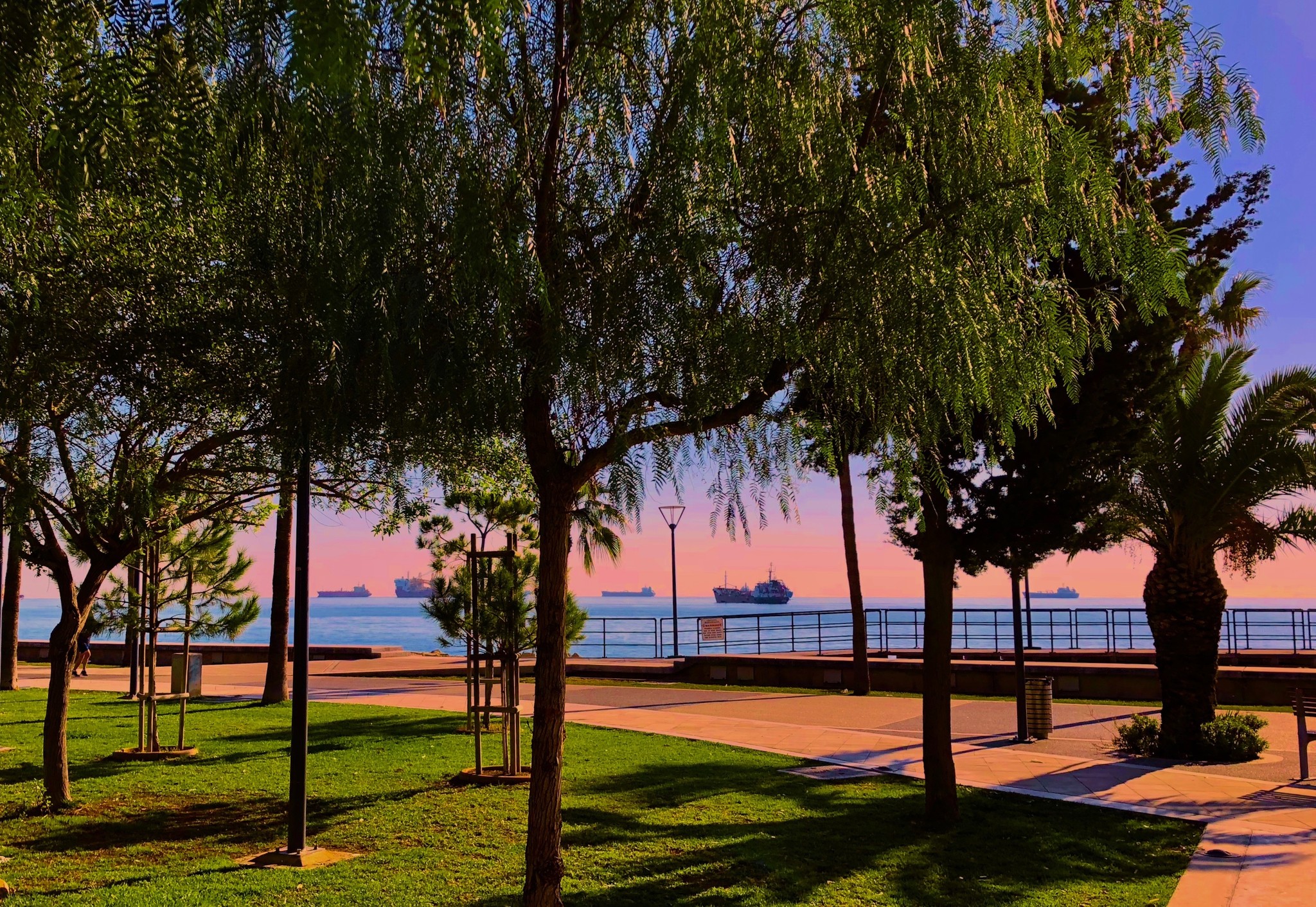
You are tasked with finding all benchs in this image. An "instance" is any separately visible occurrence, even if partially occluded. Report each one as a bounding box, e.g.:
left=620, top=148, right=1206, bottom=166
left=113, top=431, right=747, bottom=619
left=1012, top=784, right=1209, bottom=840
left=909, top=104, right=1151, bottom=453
left=1289, top=687, right=1316, bottom=779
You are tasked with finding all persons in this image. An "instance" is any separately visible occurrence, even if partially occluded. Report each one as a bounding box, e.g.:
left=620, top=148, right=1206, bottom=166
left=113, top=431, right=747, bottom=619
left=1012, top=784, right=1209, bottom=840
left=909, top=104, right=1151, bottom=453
left=72, top=630, right=92, bottom=677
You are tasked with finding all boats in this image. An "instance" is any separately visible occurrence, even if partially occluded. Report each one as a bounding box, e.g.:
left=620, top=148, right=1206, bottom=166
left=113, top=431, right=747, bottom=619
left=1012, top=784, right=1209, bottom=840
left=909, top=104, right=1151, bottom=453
left=712, top=561, right=793, bottom=605
left=601, top=586, right=656, bottom=597
left=393, top=570, right=453, bottom=598
left=317, top=581, right=372, bottom=597
left=1022, top=586, right=1080, bottom=598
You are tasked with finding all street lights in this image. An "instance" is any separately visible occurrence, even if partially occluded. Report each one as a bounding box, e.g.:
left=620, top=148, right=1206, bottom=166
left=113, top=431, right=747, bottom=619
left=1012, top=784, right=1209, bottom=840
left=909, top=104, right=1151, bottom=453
left=658, top=505, right=687, bottom=656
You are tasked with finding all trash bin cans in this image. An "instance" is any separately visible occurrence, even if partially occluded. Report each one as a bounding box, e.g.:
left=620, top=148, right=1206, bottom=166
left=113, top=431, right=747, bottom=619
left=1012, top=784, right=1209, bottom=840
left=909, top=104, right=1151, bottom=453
left=1025, top=677, right=1053, bottom=739
left=171, top=653, right=202, bottom=698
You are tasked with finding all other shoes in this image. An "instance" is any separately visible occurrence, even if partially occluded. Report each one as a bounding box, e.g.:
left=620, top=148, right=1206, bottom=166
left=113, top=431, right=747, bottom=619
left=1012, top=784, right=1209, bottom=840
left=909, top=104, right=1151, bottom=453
left=72, top=671, right=80, bottom=677
left=81, top=670, right=88, bottom=676
